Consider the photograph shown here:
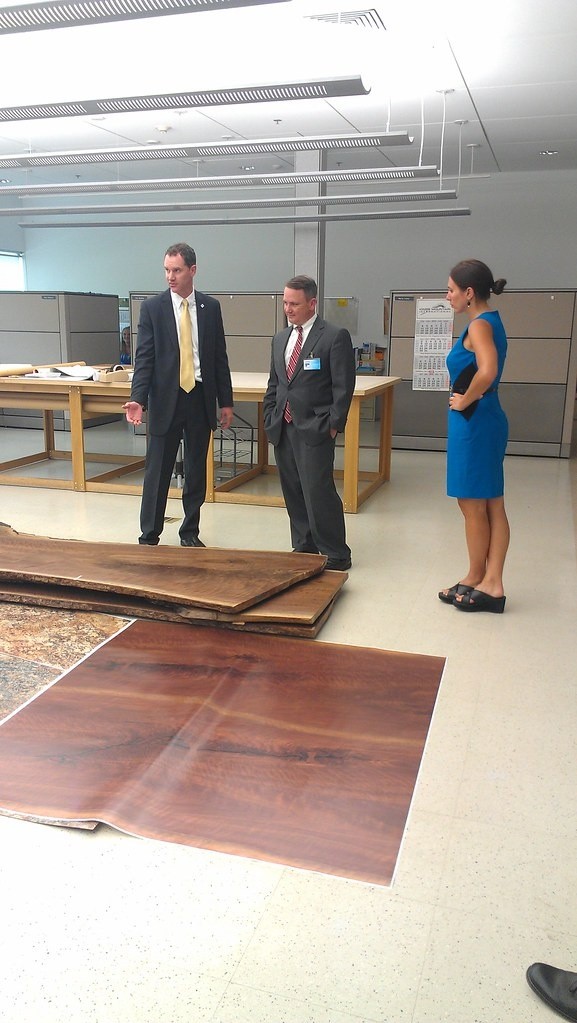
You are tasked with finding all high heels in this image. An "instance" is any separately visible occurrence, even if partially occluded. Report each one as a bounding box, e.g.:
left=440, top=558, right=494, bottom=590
left=438, top=582, right=474, bottom=604
left=452, top=590, right=505, bottom=614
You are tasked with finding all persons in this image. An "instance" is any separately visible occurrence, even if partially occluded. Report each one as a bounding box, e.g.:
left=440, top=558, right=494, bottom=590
left=262, top=276, right=352, bottom=571
left=438, top=259, right=510, bottom=613
left=120, top=325, right=131, bottom=364
left=120, top=243, right=234, bottom=547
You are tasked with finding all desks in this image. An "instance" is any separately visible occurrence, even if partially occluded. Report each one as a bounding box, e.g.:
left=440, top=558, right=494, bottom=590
left=0, top=366, right=401, bottom=514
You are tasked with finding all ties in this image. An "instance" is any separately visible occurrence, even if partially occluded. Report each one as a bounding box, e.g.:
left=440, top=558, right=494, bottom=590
left=282, top=326, right=302, bottom=424
left=179, top=299, right=195, bottom=393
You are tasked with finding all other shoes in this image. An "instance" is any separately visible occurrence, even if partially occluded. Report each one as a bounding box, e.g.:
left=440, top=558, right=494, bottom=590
left=180, top=537, right=206, bottom=547
left=291, top=549, right=319, bottom=555
left=324, top=558, right=351, bottom=572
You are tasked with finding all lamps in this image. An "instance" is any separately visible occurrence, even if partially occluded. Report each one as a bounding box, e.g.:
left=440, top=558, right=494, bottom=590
left=0, top=88, right=470, bottom=216
left=0, top=96, right=440, bottom=197
left=15, top=93, right=414, bottom=169
left=0, top=6, right=370, bottom=122
left=0, top=0, right=292, bottom=35
left=17, top=144, right=481, bottom=229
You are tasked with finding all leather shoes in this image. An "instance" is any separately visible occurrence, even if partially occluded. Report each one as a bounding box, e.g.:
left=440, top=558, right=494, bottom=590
left=525, top=962, right=577, bottom=1023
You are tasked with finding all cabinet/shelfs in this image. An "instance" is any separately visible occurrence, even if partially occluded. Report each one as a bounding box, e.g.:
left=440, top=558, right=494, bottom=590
left=356, top=371, right=383, bottom=422
